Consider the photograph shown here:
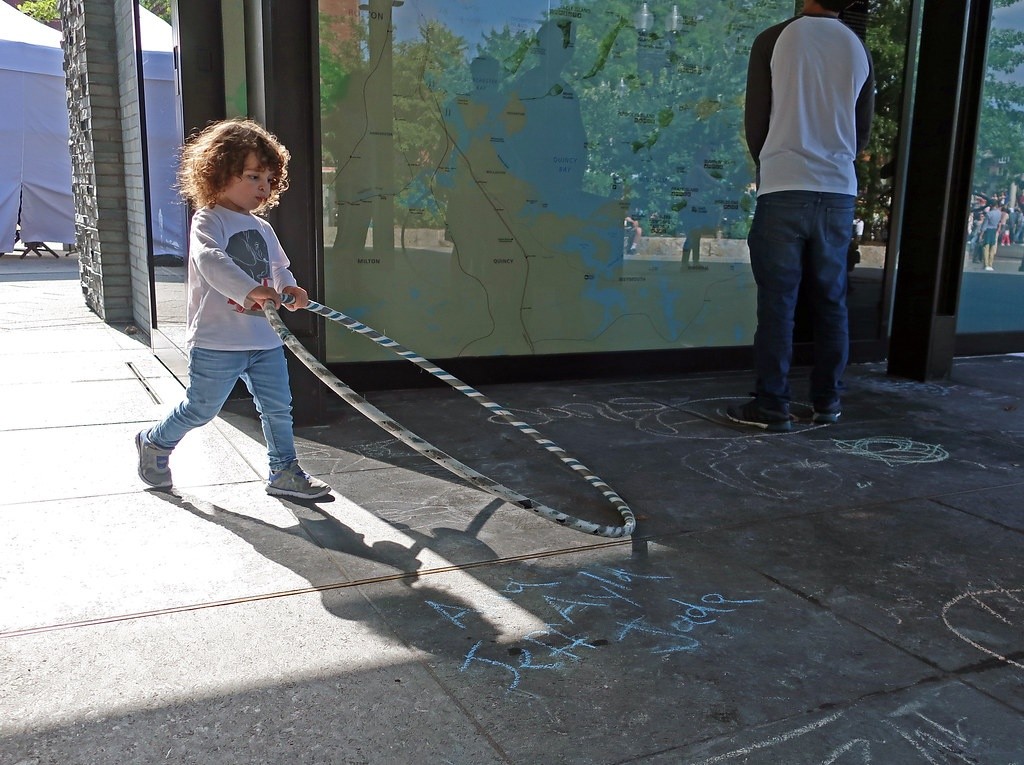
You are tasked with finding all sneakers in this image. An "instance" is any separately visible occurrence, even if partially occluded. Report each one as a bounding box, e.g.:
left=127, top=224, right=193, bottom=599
left=265, top=458, right=332, bottom=499
left=812, top=397, right=842, bottom=423
left=135, top=427, right=173, bottom=488
left=724, top=391, right=791, bottom=430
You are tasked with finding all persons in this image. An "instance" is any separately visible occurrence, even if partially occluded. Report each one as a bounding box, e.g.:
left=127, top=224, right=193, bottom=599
left=622, top=216, right=643, bottom=256
left=132, top=117, right=335, bottom=504
left=482, top=15, right=592, bottom=354
left=677, top=150, right=726, bottom=272
left=964, top=187, right=1024, bottom=271
left=721, top=0, right=878, bottom=431
left=431, top=52, right=522, bottom=310
left=326, top=69, right=403, bottom=302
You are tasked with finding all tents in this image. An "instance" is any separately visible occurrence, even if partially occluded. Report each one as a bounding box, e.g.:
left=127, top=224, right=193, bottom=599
left=138, top=5, right=187, bottom=255
left=0, top=0, right=78, bottom=258
